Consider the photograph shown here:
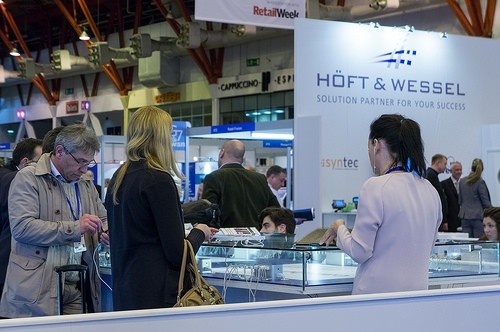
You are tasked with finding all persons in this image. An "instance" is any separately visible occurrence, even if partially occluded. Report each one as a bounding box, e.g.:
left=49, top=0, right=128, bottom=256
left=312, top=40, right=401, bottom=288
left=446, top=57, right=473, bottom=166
left=424, top=154, right=448, bottom=231
left=440, top=162, right=462, bottom=232
left=105, top=108, right=220, bottom=312
left=198, top=140, right=286, bottom=234
left=259, top=207, right=302, bottom=261
left=86, top=170, right=109, bottom=199
left=479, top=207, right=500, bottom=241
left=0, top=124, right=109, bottom=318
left=0, top=126, right=64, bottom=300
left=330, top=114, right=443, bottom=295
left=459, top=158, right=491, bottom=238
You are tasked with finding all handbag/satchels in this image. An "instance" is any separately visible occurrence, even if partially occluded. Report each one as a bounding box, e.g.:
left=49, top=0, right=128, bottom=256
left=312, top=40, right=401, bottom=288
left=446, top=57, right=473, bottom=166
left=172, top=239, right=224, bottom=307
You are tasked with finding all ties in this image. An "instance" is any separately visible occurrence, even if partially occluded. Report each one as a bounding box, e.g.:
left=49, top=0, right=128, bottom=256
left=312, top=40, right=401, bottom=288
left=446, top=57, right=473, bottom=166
left=456, top=182, right=459, bottom=194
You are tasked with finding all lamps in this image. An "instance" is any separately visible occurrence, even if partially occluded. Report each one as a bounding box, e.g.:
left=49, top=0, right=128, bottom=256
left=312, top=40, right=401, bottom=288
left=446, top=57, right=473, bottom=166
left=166, top=1, right=175, bottom=19
left=79, top=25, right=91, bottom=41
left=9, top=41, right=20, bottom=57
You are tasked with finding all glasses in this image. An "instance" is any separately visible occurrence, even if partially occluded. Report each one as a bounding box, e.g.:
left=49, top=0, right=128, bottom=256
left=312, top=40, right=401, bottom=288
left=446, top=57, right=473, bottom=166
left=64, top=147, right=97, bottom=168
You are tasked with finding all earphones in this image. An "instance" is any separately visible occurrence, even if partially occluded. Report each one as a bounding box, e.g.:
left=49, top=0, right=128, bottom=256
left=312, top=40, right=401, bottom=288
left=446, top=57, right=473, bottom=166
left=373, top=145, right=376, bottom=152
